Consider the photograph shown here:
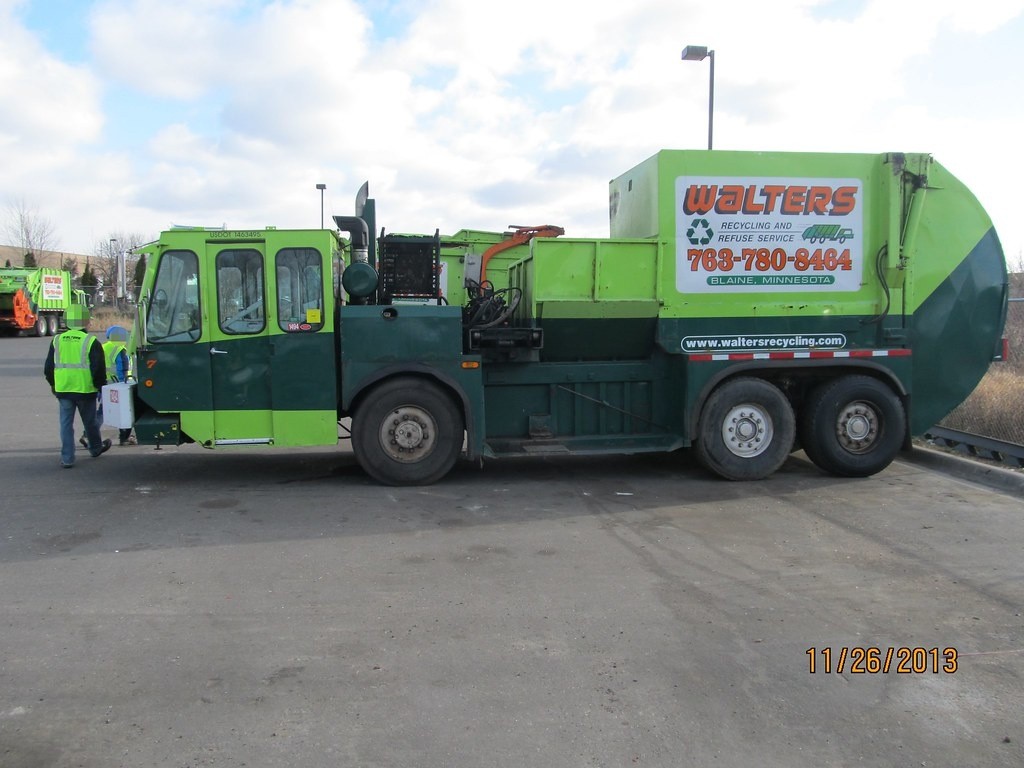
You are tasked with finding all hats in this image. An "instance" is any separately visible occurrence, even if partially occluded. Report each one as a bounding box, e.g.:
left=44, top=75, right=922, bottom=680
left=106, top=326, right=126, bottom=339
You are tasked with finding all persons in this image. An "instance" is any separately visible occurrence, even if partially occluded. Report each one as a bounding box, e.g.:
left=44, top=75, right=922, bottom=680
left=80, top=328, right=140, bottom=448
left=44, top=331, right=111, bottom=467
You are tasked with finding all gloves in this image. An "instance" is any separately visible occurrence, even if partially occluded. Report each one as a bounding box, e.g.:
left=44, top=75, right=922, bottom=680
left=52, top=385, right=55, bottom=395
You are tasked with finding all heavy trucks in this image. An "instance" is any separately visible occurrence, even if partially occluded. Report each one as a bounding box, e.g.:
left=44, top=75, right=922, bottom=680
left=0, top=264, right=93, bottom=337
left=96, top=152, right=1012, bottom=487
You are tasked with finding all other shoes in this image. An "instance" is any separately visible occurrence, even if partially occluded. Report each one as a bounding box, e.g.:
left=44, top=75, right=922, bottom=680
left=92, top=439, right=111, bottom=457
left=60, top=462, right=71, bottom=468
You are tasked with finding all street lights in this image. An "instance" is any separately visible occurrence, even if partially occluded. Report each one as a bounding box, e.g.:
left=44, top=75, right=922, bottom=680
left=108, top=237, right=118, bottom=260
left=315, top=182, right=328, bottom=229
left=681, top=46, right=717, bottom=149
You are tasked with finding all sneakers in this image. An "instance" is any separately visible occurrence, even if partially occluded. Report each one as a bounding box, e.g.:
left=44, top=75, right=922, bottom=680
left=120, top=435, right=138, bottom=445
left=79, top=436, right=88, bottom=448
